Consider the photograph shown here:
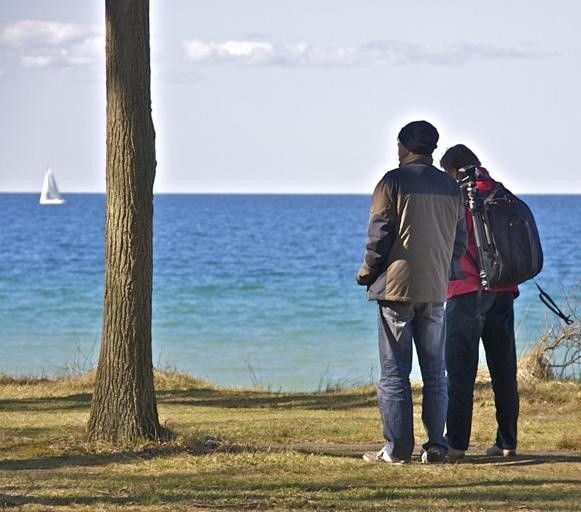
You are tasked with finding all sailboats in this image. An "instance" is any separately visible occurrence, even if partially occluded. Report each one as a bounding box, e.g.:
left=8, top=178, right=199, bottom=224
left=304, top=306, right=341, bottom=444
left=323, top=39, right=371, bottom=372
left=37, top=168, right=66, bottom=205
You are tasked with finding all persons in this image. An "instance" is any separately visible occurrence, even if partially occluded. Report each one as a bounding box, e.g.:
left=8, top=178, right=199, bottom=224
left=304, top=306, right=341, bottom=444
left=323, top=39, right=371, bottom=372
left=357, top=120, right=468, bottom=466
left=439, top=145, right=520, bottom=458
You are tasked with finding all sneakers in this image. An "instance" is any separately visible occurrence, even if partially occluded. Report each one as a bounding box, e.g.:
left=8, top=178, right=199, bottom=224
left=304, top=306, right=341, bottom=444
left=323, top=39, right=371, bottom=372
left=447, top=443, right=464, bottom=458
left=421, top=446, right=448, bottom=464
left=487, top=445, right=516, bottom=456
left=363, top=443, right=409, bottom=466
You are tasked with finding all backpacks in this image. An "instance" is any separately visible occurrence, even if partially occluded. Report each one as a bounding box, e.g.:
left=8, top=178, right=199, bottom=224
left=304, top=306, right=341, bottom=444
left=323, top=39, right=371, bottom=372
left=462, top=182, right=543, bottom=288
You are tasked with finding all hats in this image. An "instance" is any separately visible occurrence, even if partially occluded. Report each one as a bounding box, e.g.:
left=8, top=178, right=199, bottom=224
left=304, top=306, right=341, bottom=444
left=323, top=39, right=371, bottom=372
left=399, top=121, right=439, bottom=154
